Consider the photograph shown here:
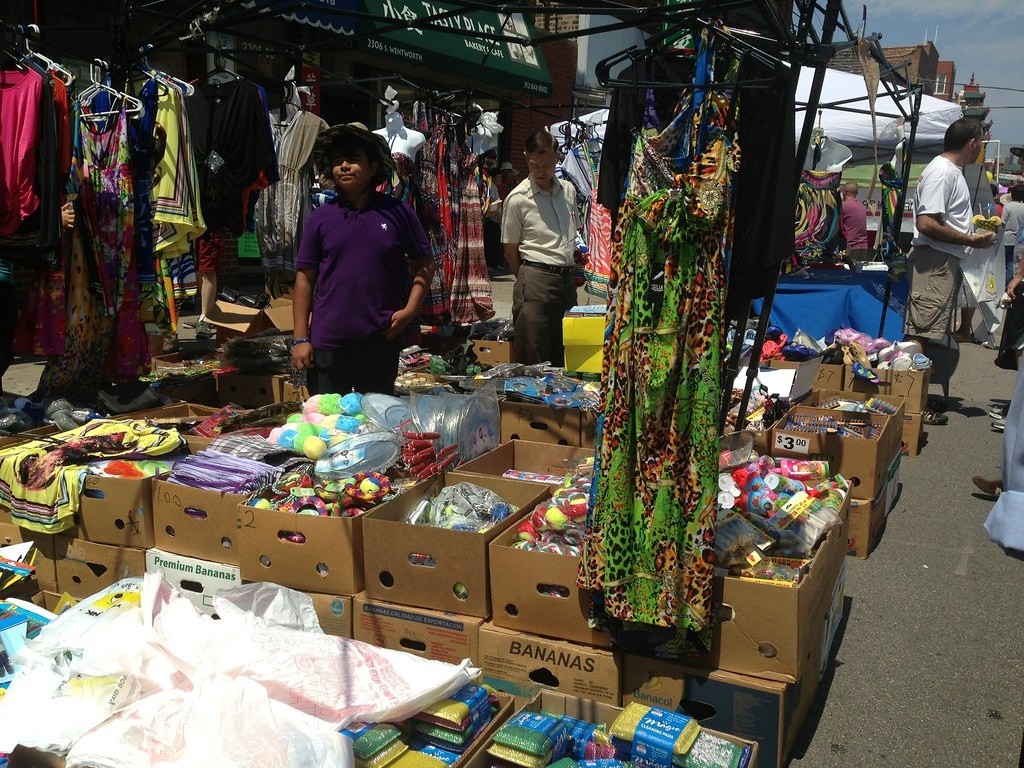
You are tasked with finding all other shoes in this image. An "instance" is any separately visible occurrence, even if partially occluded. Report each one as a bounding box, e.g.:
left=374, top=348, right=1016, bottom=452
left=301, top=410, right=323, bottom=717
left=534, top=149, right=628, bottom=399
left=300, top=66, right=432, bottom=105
left=989, top=407, right=1006, bottom=419
left=992, top=420, right=1006, bottom=429
left=182, top=320, right=211, bottom=329
left=973, top=475, right=1003, bottom=496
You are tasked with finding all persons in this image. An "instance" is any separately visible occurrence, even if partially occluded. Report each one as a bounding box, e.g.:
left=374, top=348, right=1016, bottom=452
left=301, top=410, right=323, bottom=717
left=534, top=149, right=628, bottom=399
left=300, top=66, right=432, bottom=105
left=902, top=119, right=999, bottom=425
left=292, top=123, right=436, bottom=398
left=841, top=182, right=869, bottom=261
left=182, top=235, right=227, bottom=339
left=501, top=132, right=578, bottom=368
left=950, top=178, right=1024, bottom=429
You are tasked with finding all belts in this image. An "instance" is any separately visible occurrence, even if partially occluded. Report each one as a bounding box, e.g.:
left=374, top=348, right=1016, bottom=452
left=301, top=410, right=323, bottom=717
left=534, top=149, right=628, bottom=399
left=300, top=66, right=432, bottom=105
left=523, top=260, right=576, bottom=275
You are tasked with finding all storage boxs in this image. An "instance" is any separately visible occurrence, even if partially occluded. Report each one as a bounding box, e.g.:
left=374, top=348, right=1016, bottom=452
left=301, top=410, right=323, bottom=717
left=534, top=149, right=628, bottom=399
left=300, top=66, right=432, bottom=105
left=213, top=373, right=292, bottom=410
left=283, top=381, right=312, bottom=404
left=151, top=352, right=196, bottom=374
left=107, top=401, right=223, bottom=436
left=0, top=422, right=82, bottom=613
left=53, top=531, right=146, bottom=603
left=176, top=315, right=217, bottom=352
left=151, top=471, right=259, bottom=566
left=145, top=548, right=242, bottom=615
left=235, top=472, right=440, bottom=597
left=61, top=441, right=210, bottom=549
left=351, top=308, right=934, bottom=768
left=301, top=592, right=356, bottom=640
left=201, top=296, right=312, bottom=348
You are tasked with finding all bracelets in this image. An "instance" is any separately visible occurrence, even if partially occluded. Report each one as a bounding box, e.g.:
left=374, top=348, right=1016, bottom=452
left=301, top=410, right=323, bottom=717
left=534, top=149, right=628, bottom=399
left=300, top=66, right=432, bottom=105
left=293, top=338, right=310, bottom=344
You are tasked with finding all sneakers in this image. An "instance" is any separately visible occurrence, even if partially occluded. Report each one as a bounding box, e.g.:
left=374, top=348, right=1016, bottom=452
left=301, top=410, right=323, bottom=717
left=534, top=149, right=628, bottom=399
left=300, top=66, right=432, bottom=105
left=923, top=408, right=948, bottom=424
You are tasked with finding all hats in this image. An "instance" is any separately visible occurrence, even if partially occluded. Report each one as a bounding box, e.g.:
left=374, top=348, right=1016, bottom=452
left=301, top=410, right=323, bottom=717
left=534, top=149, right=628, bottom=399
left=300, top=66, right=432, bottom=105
left=314, top=122, right=393, bottom=190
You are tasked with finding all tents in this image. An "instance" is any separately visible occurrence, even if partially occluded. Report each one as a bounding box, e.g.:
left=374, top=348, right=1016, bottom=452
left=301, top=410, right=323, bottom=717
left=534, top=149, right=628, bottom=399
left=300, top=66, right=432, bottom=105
left=550, top=60, right=962, bottom=166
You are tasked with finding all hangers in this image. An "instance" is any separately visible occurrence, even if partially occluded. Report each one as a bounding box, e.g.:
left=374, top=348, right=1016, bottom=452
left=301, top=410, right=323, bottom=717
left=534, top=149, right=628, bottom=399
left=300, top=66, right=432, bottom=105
left=553, top=114, right=605, bottom=158
left=0, top=19, right=303, bottom=128
left=402, top=84, right=463, bottom=143
left=594, top=7, right=794, bottom=90
